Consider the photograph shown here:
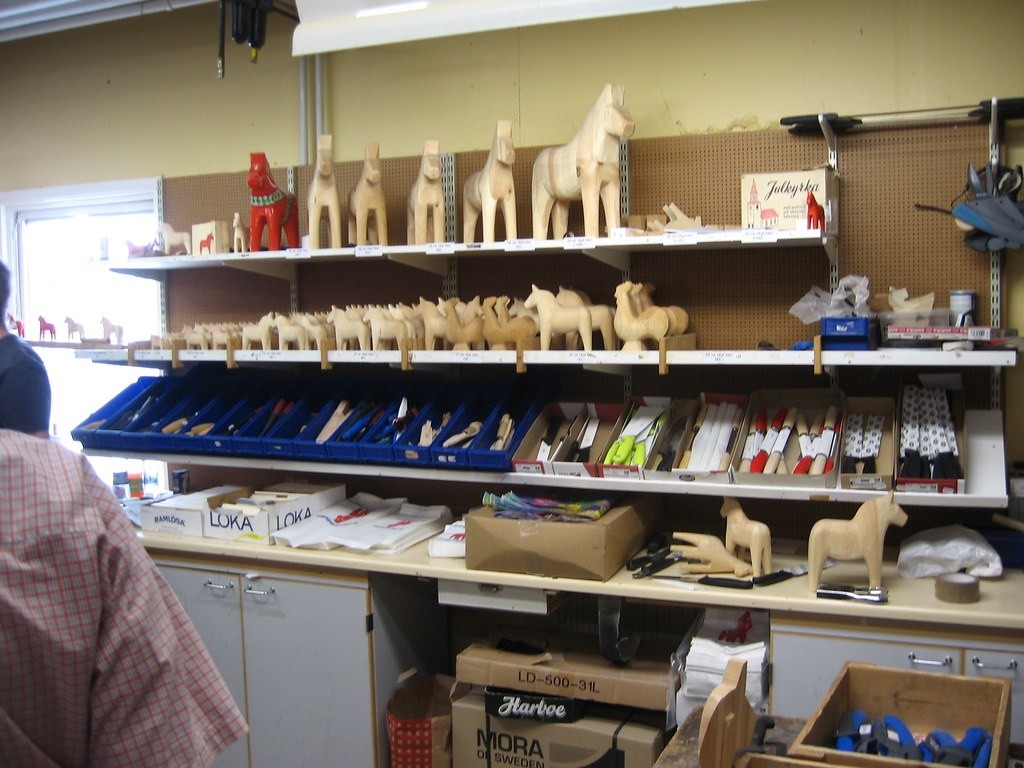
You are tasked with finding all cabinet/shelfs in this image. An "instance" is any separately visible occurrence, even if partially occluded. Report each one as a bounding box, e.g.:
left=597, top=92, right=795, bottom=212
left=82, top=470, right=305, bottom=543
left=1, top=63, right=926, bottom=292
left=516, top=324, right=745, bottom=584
left=71, top=95, right=1023, bottom=632
left=152, top=564, right=377, bottom=768
left=769, top=610, right=1023, bottom=743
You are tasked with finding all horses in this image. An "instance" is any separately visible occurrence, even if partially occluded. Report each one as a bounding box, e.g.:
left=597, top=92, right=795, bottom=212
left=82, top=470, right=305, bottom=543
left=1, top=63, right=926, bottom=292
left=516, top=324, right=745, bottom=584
left=126, top=211, right=249, bottom=256
left=807, top=488, right=910, bottom=593
left=5, top=278, right=690, bottom=375
left=646, top=200, right=703, bottom=234
left=531, top=80, right=637, bottom=241
left=306, top=117, right=517, bottom=250
left=248, top=152, right=300, bottom=251
left=671, top=491, right=776, bottom=589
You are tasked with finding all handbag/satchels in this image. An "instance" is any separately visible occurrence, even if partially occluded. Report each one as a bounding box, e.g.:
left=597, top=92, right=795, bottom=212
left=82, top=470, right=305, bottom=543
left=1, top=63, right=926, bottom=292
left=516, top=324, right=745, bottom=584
left=386, top=666, right=457, bottom=768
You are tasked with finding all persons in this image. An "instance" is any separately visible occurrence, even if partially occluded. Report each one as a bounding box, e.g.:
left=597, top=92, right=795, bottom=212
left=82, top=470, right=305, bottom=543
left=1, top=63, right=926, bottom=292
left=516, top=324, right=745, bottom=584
left=0, top=262, right=247, bottom=766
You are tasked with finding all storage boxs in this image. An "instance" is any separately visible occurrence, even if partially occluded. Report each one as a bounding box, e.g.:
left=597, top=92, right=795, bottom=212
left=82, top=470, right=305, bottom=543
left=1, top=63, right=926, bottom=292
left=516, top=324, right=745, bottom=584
left=71, top=365, right=1013, bottom=768
left=819, top=316, right=880, bottom=351
left=876, top=309, right=952, bottom=349
left=739, top=170, right=839, bottom=238
